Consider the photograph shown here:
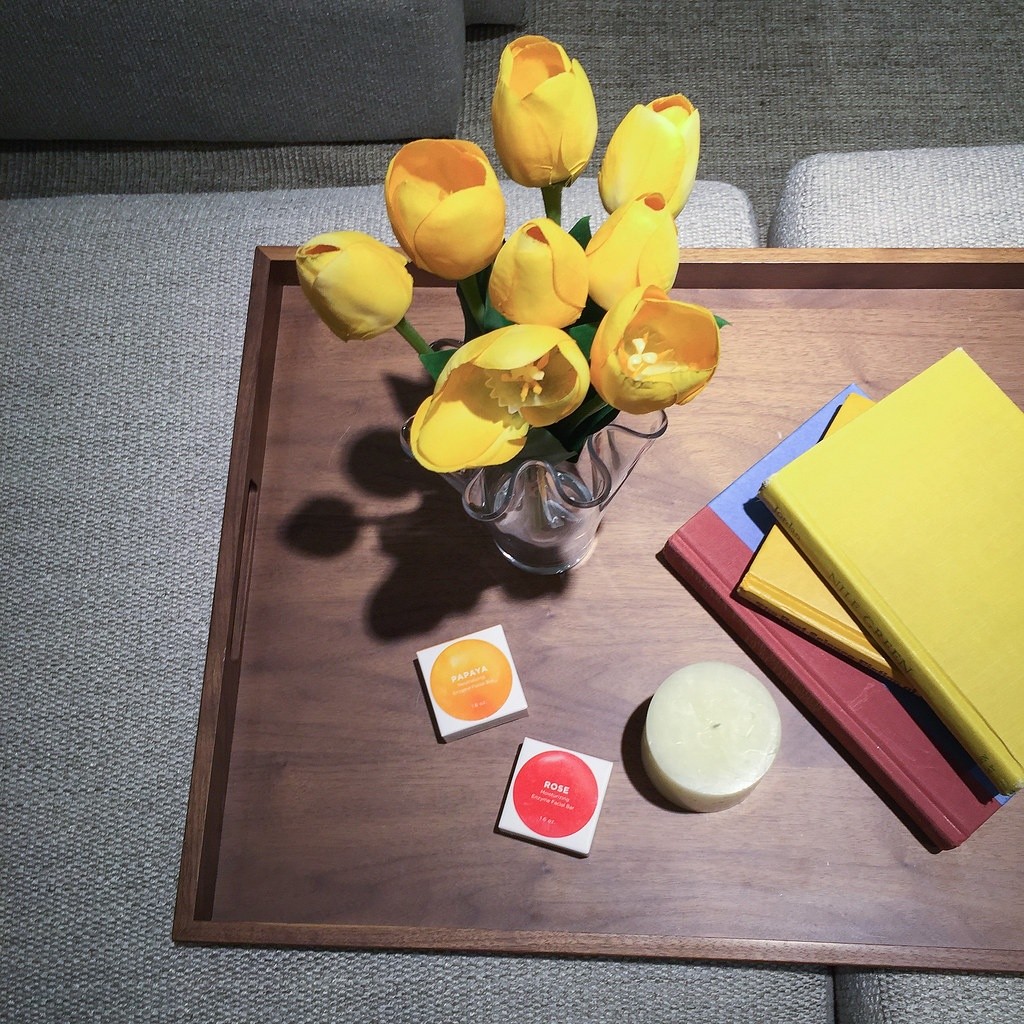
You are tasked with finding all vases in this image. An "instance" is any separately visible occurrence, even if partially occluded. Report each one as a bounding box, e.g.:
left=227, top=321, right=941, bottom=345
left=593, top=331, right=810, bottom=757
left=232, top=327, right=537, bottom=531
left=400, top=338, right=669, bottom=573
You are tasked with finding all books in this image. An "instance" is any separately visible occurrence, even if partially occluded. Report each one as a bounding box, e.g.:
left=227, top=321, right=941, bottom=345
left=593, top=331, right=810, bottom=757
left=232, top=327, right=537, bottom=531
left=662, top=347, right=1024, bottom=850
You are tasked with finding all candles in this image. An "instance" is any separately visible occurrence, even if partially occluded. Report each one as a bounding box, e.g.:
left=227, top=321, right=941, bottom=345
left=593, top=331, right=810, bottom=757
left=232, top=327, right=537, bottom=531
left=642, top=661, right=782, bottom=815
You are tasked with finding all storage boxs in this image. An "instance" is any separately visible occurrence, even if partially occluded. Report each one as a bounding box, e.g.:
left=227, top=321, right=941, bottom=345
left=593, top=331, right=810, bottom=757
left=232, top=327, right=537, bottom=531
left=415, top=623, right=530, bottom=744
left=498, top=736, right=614, bottom=857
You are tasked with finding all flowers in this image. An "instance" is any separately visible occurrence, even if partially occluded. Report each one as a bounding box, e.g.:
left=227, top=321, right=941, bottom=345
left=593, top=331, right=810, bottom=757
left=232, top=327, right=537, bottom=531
left=295, top=35, right=720, bottom=474
